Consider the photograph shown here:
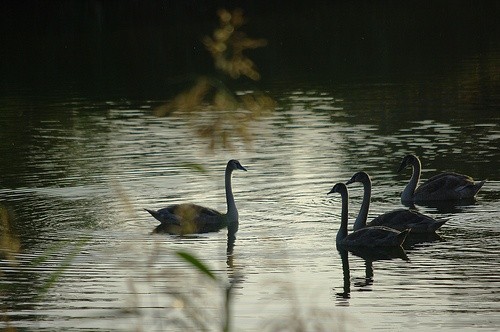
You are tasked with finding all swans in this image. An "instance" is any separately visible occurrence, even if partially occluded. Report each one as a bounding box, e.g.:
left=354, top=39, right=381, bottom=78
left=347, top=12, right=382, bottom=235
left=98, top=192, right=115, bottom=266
left=346, top=172, right=451, bottom=231
left=142, top=159, right=248, bottom=225
left=399, top=155, right=488, bottom=204
left=336, top=245, right=410, bottom=299
left=327, top=183, right=411, bottom=247
